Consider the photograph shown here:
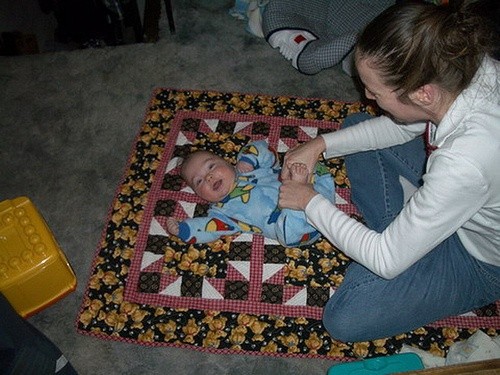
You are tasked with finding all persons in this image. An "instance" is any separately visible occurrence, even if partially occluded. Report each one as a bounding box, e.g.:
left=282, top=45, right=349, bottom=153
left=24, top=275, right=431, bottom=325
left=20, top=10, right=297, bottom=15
left=278, top=0, right=500, bottom=341
left=166, top=140, right=336, bottom=247
